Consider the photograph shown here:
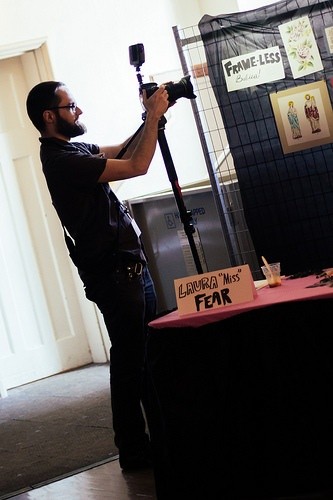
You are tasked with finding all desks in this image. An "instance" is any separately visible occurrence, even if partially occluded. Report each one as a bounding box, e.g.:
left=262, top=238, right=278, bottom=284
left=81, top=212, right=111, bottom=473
left=147, top=267, right=333, bottom=429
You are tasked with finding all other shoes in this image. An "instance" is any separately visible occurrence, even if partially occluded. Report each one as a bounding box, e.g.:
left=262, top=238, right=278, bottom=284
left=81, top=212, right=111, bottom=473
left=121, top=441, right=153, bottom=473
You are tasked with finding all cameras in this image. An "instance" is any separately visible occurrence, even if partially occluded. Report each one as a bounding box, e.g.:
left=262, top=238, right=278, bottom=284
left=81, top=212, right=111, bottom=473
left=139, top=75, right=197, bottom=99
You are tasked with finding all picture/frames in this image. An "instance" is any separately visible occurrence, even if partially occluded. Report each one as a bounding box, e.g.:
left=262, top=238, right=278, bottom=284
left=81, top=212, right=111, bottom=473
left=269, top=80, right=333, bottom=155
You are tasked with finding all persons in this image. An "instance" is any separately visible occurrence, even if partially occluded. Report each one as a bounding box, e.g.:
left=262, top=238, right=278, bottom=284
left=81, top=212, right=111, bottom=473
left=26, top=81, right=170, bottom=469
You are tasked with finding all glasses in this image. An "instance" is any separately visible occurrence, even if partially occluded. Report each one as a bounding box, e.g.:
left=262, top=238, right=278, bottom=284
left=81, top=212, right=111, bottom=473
left=49, top=103, right=77, bottom=111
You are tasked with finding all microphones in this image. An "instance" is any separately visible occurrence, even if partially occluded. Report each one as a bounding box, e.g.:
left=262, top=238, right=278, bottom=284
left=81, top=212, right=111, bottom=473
left=129, top=43, right=145, bottom=84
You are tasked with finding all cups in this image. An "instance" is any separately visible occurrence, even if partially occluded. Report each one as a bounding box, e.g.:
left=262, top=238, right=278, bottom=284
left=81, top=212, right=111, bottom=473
left=261, top=262, right=281, bottom=288
